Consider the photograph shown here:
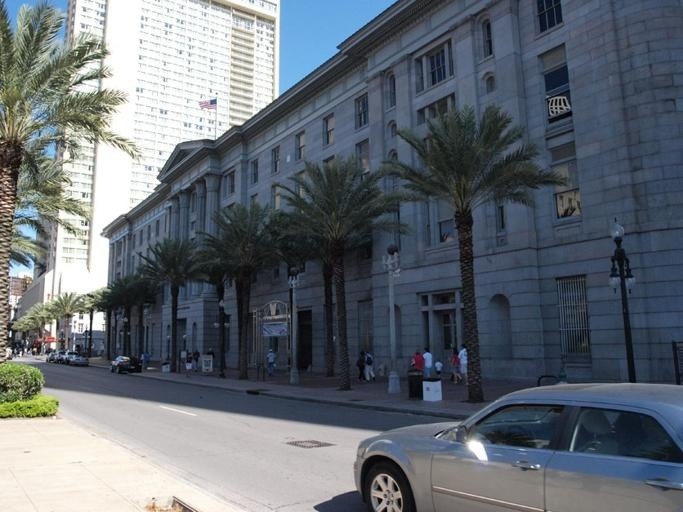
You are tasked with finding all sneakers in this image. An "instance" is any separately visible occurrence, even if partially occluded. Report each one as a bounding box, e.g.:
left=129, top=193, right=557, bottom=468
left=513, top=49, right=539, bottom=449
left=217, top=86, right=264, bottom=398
left=357, top=376, right=375, bottom=385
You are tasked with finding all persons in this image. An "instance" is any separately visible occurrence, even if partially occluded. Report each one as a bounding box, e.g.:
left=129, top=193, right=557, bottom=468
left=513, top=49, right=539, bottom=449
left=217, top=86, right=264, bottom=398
left=448, top=348, right=463, bottom=385
left=207, top=347, right=214, bottom=360
left=193, top=349, right=200, bottom=372
left=144, top=351, right=152, bottom=370
left=433, top=359, right=444, bottom=377
left=139, top=351, right=144, bottom=369
left=265, top=348, right=275, bottom=377
left=355, top=351, right=365, bottom=382
left=364, top=351, right=376, bottom=382
left=409, top=352, right=424, bottom=371
left=457, top=343, right=469, bottom=386
left=184, top=353, right=193, bottom=378
left=421, top=346, right=432, bottom=378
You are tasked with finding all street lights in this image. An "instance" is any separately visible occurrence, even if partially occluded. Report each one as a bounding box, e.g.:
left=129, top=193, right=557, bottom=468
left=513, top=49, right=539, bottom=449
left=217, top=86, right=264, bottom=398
left=608, top=219, right=635, bottom=384
left=61, top=329, right=64, bottom=346
left=121, top=317, right=131, bottom=358
left=213, top=300, right=229, bottom=379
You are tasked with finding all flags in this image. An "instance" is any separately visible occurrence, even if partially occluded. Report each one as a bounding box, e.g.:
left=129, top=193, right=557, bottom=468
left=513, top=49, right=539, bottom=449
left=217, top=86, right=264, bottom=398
left=198, top=98, right=217, bottom=112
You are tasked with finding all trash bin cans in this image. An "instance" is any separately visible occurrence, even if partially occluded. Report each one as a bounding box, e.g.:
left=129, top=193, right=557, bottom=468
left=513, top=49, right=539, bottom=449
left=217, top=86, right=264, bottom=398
left=407, top=368, right=442, bottom=401
left=161, top=362, right=169, bottom=372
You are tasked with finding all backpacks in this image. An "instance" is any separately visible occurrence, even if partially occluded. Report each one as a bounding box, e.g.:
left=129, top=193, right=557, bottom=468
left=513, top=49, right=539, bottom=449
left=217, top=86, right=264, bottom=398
left=365, top=354, right=372, bottom=366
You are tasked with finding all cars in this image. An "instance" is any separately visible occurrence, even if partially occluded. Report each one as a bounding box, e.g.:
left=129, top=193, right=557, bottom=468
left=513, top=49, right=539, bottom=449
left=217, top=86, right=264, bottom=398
left=45, top=349, right=89, bottom=366
left=352, top=382, right=683, bottom=512
left=112, top=354, right=142, bottom=374
